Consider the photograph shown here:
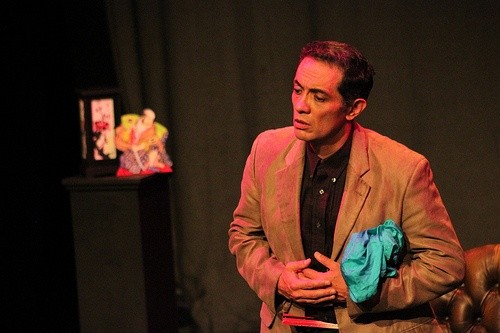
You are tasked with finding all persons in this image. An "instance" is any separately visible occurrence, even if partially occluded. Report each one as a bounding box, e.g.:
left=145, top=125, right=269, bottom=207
left=229, top=40, right=465, bottom=332
